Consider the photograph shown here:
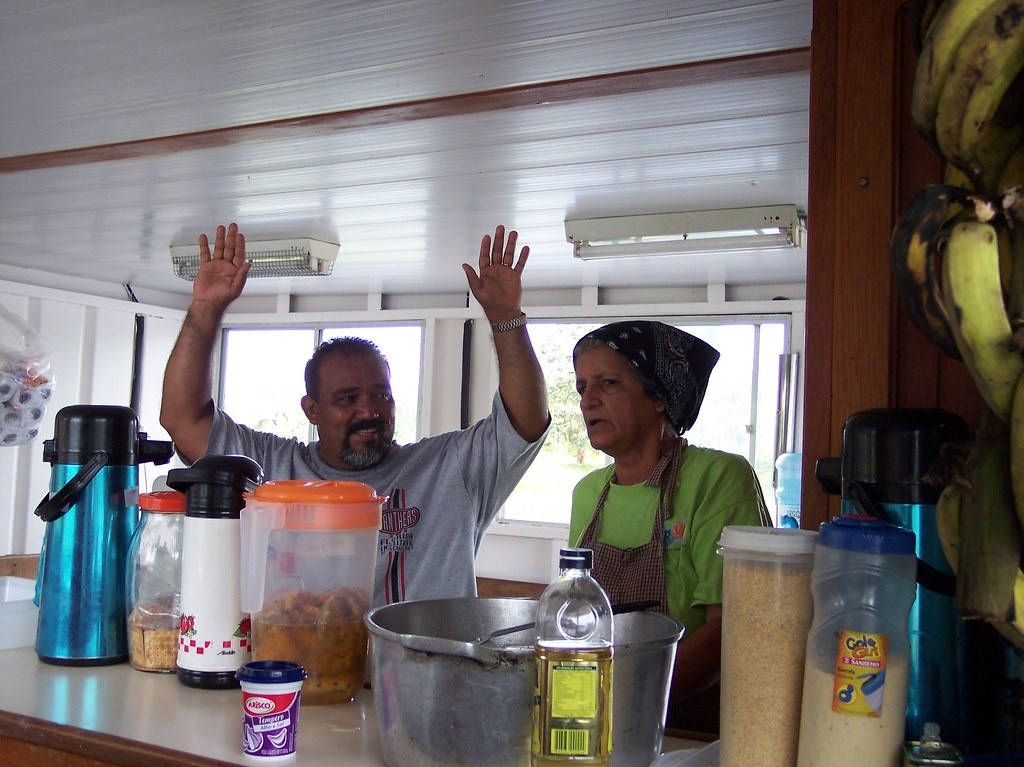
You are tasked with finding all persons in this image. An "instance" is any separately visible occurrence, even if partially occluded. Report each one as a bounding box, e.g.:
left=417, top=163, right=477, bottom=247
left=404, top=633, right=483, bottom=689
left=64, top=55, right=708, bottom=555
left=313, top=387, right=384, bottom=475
left=158, top=219, right=555, bottom=613
left=567, top=320, right=778, bottom=739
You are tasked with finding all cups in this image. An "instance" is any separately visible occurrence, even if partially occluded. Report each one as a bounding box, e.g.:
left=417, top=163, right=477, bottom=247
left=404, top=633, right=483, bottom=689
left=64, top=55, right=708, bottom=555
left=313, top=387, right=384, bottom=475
left=235, top=661, right=307, bottom=761
left=238, top=482, right=389, bottom=707
left=714, top=526, right=820, bottom=767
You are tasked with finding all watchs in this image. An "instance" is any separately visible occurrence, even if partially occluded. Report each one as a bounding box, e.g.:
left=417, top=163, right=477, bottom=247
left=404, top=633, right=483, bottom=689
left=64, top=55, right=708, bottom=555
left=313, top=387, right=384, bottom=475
left=491, top=311, right=528, bottom=333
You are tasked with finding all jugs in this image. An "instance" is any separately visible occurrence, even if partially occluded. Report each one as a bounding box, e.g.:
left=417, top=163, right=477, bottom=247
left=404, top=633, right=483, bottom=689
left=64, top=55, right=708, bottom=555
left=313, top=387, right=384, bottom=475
left=165, top=455, right=264, bottom=690
left=815, top=411, right=974, bottom=743
left=38, top=404, right=173, bottom=667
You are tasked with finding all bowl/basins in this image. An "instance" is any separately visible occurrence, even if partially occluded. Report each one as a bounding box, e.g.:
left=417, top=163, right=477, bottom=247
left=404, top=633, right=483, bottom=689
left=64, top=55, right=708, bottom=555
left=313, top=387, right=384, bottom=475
left=364, top=599, right=685, bottom=767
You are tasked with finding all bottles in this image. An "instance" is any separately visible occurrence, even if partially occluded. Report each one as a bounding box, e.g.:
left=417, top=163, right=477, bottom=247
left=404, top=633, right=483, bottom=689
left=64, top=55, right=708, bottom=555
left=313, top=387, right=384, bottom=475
left=797, top=520, right=920, bottom=767
left=775, top=452, right=801, bottom=529
left=125, top=492, right=184, bottom=673
left=531, top=549, right=614, bottom=767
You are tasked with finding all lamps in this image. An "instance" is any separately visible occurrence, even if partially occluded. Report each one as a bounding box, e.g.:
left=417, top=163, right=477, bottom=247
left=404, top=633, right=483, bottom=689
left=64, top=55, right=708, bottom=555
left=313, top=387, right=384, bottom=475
left=166, top=237, right=341, bottom=282
left=563, top=203, right=802, bottom=262
